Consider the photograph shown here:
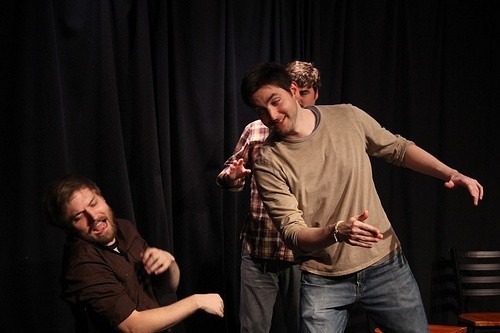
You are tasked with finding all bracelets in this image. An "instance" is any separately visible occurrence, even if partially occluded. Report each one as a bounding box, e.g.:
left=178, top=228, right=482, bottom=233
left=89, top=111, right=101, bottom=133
left=333, top=221, right=344, bottom=244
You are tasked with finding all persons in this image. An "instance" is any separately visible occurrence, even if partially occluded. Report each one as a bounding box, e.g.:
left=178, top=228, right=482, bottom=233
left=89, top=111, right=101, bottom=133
left=54, top=173, right=225, bottom=333
left=241, top=67, right=484, bottom=333
left=217, top=61, right=319, bottom=333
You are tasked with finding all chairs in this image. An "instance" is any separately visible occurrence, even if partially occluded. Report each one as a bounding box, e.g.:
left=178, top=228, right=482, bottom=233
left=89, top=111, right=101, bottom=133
left=449, top=247, right=500, bottom=333
left=71, top=303, right=106, bottom=333
left=367, top=319, right=469, bottom=333
